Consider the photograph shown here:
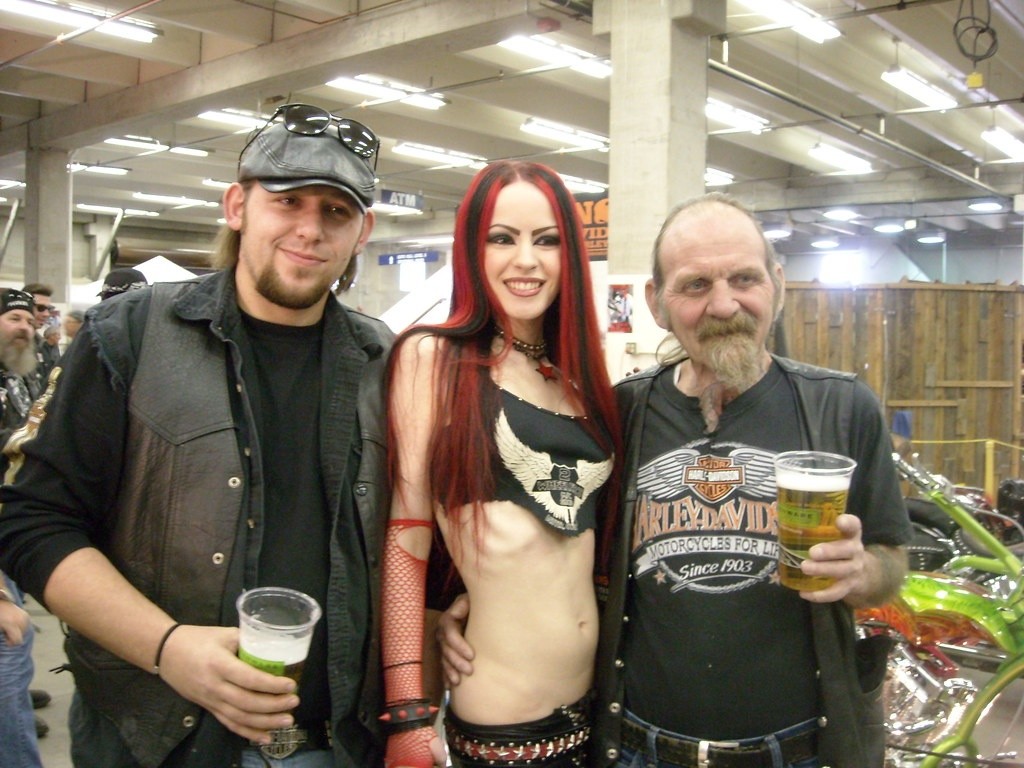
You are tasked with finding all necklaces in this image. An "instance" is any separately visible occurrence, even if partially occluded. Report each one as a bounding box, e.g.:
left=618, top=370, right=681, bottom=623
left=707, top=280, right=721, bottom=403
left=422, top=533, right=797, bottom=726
left=493, top=326, right=558, bottom=381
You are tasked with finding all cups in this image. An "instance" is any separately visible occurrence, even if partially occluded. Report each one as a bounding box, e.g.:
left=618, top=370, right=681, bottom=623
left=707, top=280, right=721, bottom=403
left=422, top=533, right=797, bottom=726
left=774, top=451, right=856, bottom=592
left=236, top=590, right=321, bottom=697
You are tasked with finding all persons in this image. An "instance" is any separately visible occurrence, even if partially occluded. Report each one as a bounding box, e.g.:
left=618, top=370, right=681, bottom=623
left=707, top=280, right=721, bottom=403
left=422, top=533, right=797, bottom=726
left=0, top=269, right=147, bottom=768
left=0, top=121, right=396, bottom=768
left=381, top=155, right=622, bottom=768
left=435, top=191, right=914, bottom=768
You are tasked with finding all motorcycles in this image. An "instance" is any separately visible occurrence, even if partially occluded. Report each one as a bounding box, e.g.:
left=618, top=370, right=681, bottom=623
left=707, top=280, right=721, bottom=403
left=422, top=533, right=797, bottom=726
left=852, top=452, right=1024, bottom=768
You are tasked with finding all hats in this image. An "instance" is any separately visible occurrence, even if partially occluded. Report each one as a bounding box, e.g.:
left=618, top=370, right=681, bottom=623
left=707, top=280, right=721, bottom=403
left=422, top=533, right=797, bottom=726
left=67, top=310, right=87, bottom=323
left=96, top=268, right=149, bottom=300
left=0, top=287, right=34, bottom=314
left=238, top=121, right=377, bottom=216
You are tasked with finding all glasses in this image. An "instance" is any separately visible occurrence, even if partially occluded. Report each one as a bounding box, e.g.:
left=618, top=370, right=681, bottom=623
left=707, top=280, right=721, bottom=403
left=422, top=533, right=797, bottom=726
left=34, top=303, right=55, bottom=313
left=238, top=102, right=380, bottom=172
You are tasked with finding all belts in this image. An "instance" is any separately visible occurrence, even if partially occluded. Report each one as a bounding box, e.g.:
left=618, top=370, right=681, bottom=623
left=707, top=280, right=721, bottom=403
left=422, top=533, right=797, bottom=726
left=622, top=719, right=821, bottom=767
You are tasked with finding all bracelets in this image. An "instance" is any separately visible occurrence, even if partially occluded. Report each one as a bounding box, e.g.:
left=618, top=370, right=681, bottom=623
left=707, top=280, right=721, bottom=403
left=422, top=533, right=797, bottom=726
left=383, top=660, right=422, bottom=670
left=378, top=698, right=440, bottom=736
left=155, top=623, right=181, bottom=678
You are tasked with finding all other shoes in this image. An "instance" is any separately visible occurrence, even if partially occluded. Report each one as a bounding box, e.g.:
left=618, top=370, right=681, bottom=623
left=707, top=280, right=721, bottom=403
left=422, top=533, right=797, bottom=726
left=34, top=715, right=48, bottom=737
left=28, top=689, right=52, bottom=708
